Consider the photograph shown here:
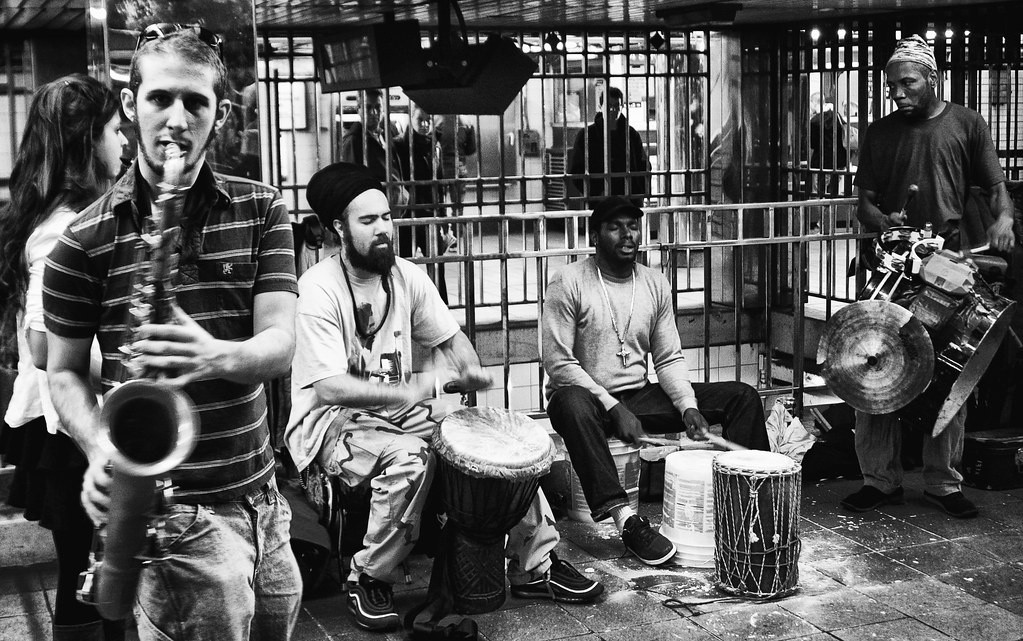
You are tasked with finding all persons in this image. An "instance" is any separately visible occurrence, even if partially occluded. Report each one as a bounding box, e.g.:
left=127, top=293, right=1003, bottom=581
left=810, top=91, right=855, bottom=232
left=40, top=29, right=303, bottom=641
left=0, top=73, right=129, bottom=641
left=391, top=101, right=476, bottom=291
left=539, top=194, right=774, bottom=569
left=284, top=160, right=607, bottom=632
left=572, top=85, right=654, bottom=258
left=840, top=33, right=1015, bottom=520
left=339, top=88, right=410, bottom=220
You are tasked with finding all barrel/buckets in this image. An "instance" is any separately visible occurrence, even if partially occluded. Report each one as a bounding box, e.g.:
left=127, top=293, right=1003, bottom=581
left=560, top=437, right=642, bottom=523
left=658, top=448, right=726, bottom=569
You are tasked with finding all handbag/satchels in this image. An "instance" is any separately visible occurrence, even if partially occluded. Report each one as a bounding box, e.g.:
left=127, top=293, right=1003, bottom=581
left=842, top=124, right=859, bottom=149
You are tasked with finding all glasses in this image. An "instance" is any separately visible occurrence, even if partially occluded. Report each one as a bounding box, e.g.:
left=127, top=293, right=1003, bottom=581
left=136, top=21, right=225, bottom=64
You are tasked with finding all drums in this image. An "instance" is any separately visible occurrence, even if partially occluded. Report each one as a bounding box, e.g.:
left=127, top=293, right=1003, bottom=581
left=427, top=405, right=558, bottom=617
left=855, top=241, right=1017, bottom=439
left=711, top=449, right=803, bottom=601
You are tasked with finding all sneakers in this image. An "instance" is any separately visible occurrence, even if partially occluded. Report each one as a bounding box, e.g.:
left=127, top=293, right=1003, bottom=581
left=620, top=514, right=676, bottom=566
left=347, top=581, right=399, bottom=628
left=512, top=550, right=604, bottom=603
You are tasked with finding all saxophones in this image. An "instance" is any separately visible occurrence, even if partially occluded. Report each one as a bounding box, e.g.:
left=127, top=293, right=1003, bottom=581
left=71, top=141, right=207, bottom=622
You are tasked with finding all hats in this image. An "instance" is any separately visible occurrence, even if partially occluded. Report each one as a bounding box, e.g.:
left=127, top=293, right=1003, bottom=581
left=306, top=161, right=386, bottom=234
left=590, top=195, right=644, bottom=230
left=887, top=34, right=937, bottom=71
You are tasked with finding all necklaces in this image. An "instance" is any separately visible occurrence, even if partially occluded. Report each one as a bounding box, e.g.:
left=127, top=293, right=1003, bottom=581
left=337, top=248, right=391, bottom=349
left=594, top=258, right=637, bottom=370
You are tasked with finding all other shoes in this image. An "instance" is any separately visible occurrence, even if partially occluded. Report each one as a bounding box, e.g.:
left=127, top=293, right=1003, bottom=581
left=924, top=489, right=978, bottom=517
left=840, top=484, right=904, bottom=511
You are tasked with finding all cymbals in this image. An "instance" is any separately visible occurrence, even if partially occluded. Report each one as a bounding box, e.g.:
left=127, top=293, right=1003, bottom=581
left=814, top=299, right=936, bottom=416
left=872, top=222, right=945, bottom=275
